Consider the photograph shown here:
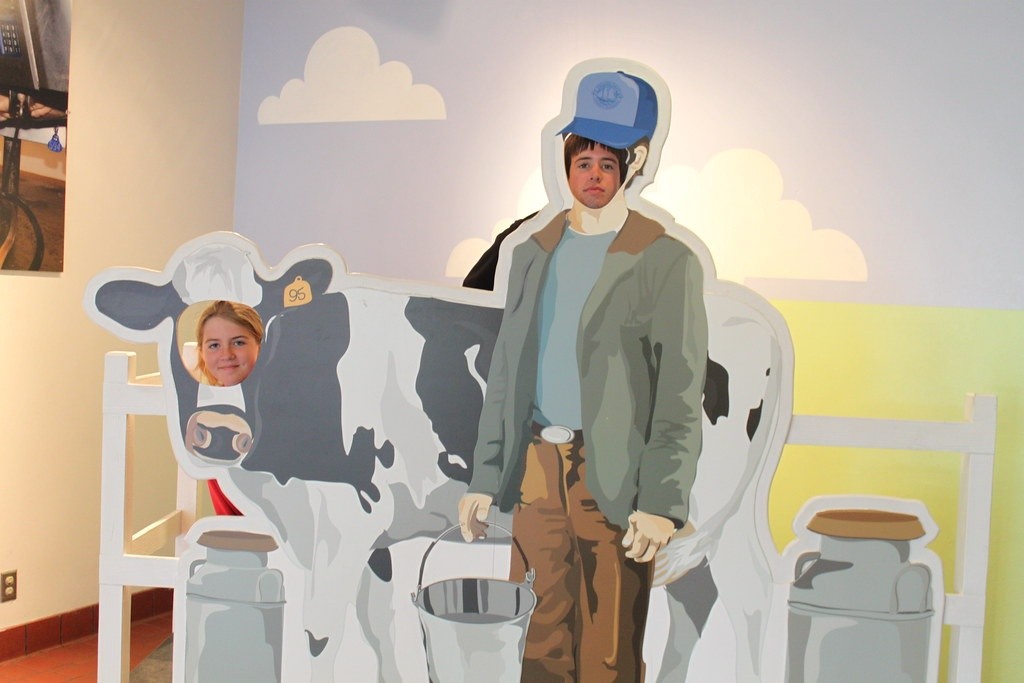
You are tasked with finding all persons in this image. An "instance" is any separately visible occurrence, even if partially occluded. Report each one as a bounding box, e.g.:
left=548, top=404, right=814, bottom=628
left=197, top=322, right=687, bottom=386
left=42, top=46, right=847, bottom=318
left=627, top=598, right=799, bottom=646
left=196, top=300, right=265, bottom=388
left=563, top=134, right=629, bottom=209
